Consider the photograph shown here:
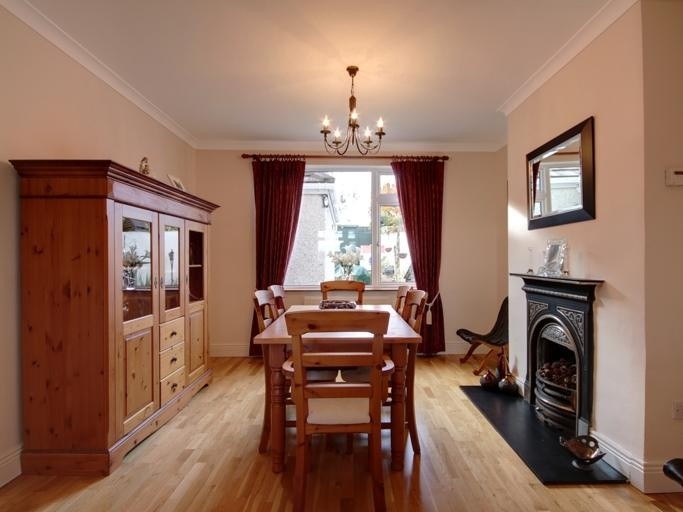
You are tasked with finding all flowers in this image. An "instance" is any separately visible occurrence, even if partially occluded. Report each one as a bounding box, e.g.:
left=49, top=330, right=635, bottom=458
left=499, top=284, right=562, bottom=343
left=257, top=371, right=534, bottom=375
left=326, top=242, right=364, bottom=281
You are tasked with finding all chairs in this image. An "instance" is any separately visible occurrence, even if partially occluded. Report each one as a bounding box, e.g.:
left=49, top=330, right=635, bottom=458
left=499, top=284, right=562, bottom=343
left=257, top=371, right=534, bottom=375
left=391, top=285, right=411, bottom=316
left=455, top=295, right=507, bottom=379
left=250, top=287, right=339, bottom=453
left=341, top=288, right=428, bottom=458
left=268, top=282, right=286, bottom=318
left=318, top=281, right=365, bottom=306
left=283, top=310, right=390, bottom=470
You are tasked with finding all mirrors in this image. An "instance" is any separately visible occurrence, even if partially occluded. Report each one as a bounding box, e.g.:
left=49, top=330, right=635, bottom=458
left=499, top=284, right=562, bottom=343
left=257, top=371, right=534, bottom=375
left=524, top=116, right=596, bottom=231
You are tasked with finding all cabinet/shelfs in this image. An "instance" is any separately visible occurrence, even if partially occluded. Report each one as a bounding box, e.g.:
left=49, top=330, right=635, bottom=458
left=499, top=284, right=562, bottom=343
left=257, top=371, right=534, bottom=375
left=9, top=159, right=222, bottom=478
left=318, top=64, right=385, bottom=156
